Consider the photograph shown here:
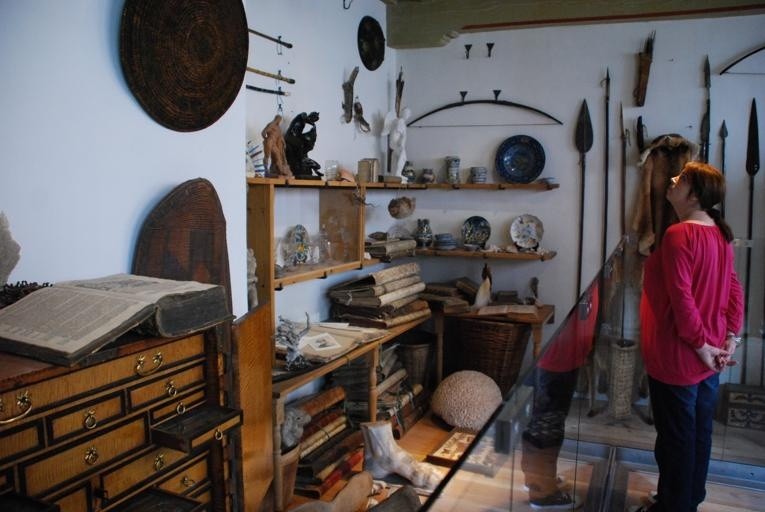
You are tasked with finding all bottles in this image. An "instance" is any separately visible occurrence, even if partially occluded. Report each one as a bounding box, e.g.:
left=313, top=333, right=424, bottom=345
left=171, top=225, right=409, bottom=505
left=403, top=161, right=415, bottom=183
left=317, top=224, right=333, bottom=263
left=421, top=168, right=435, bottom=183
left=444, top=156, right=461, bottom=184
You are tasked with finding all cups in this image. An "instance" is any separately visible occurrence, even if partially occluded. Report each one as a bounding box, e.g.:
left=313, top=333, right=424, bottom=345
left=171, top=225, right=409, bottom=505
left=324, top=160, right=339, bottom=180
left=464, top=244, right=479, bottom=251
left=471, top=167, right=487, bottom=184
left=416, top=218, right=432, bottom=250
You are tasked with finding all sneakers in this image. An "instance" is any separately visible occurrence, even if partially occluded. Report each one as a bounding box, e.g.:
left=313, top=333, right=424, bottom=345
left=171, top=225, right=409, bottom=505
left=629, top=490, right=657, bottom=512
left=523, top=476, right=582, bottom=509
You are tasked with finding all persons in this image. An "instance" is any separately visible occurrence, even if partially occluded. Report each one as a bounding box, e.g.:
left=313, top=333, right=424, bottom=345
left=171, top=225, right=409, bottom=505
left=261, top=115, right=287, bottom=174
left=520, top=276, right=599, bottom=505
left=638, top=159, right=746, bottom=512
left=285, top=110, right=321, bottom=169
left=390, top=106, right=412, bottom=179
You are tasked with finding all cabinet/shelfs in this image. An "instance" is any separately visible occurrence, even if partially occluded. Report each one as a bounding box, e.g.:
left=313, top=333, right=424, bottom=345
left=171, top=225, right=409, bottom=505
left=244, top=176, right=556, bottom=512
left=0, top=309, right=243, bottom=511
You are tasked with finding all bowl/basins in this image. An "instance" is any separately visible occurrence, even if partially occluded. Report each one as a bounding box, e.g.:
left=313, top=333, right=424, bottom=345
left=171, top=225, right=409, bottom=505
left=434, top=233, right=458, bottom=250
left=544, top=178, right=555, bottom=183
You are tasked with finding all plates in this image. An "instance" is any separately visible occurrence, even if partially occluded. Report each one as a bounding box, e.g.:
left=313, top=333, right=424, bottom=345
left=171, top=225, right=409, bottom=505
left=290, top=225, right=310, bottom=262
left=461, top=216, right=490, bottom=244
left=494, top=134, right=546, bottom=183
left=509, top=214, right=544, bottom=249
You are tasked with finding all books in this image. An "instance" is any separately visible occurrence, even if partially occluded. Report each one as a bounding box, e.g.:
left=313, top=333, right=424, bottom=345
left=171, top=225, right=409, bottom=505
left=0, top=272, right=237, bottom=368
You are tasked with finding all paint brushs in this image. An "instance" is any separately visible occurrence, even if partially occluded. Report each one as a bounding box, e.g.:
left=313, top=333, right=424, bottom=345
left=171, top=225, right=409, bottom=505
left=246, top=85, right=290, bottom=96
left=248, top=28, right=292, bottom=48
left=246, top=65, right=295, bottom=84
